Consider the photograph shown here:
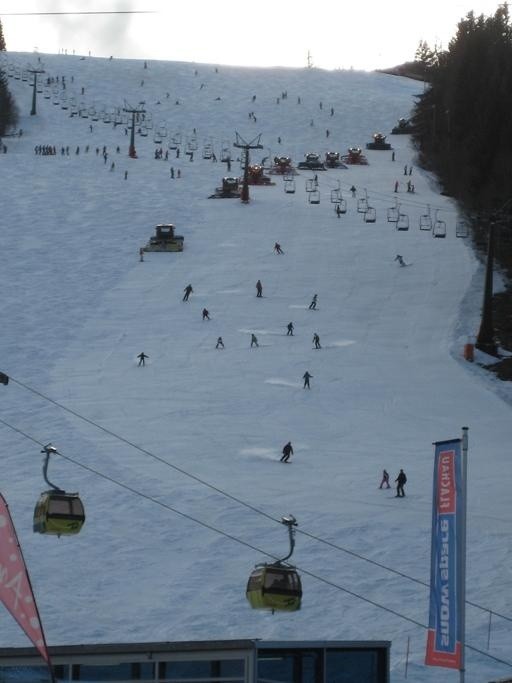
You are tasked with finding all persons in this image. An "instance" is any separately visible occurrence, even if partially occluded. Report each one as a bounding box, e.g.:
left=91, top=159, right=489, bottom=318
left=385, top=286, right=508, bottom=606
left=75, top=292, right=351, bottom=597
left=394, top=253, right=406, bottom=266
left=314, top=151, right=414, bottom=196
left=280, top=440, right=294, bottom=463
left=394, top=468, right=407, bottom=497
left=280, top=577, right=288, bottom=589
left=136, top=114, right=240, bottom=179
left=135, top=276, right=321, bottom=389
left=274, top=241, right=284, bottom=255
left=20, top=120, right=129, bottom=180
left=248, top=90, right=334, bottom=142
left=139, top=61, right=221, bottom=104
left=47, top=75, right=84, bottom=95
left=379, top=469, right=390, bottom=489
left=270, top=578, right=280, bottom=588
